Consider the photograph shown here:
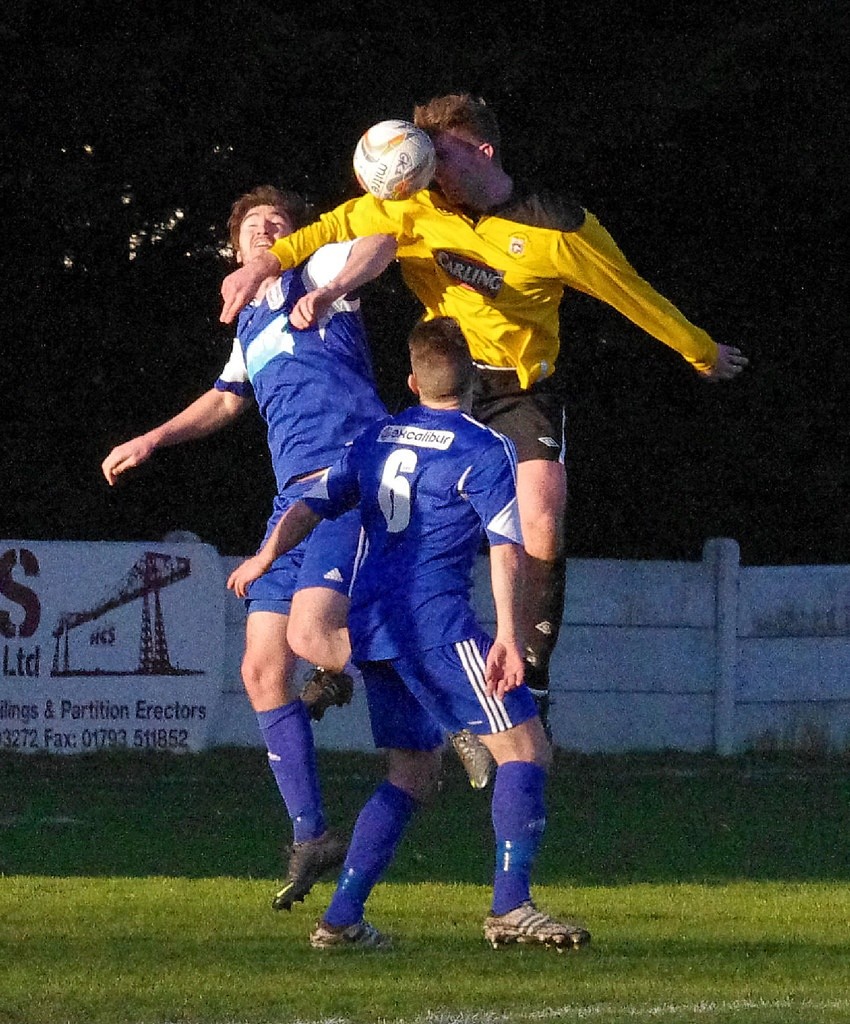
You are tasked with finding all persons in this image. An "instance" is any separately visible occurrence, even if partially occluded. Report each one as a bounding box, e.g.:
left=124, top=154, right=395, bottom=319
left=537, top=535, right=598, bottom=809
left=99, top=183, right=402, bottom=919
left=218, top=92, right=751, bottom=794
left=223, top=313, right=593, bottom=953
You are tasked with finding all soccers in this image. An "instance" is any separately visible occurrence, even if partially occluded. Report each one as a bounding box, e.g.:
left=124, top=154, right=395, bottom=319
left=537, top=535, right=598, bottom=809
left=352, top=119, right=438, bottom=202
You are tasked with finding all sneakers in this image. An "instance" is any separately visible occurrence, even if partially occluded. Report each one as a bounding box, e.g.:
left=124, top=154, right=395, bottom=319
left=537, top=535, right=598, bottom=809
left=300, top=667, right=355, bottom=722
left=272, top=825, right=352, bottom=914
left=480, top=901, right=590, bottom=954
left=308, top=918, right=394, bottom=952
left=446, top=729, right=498, bottom=789
left=521, top=657, right=553, bottom=748
